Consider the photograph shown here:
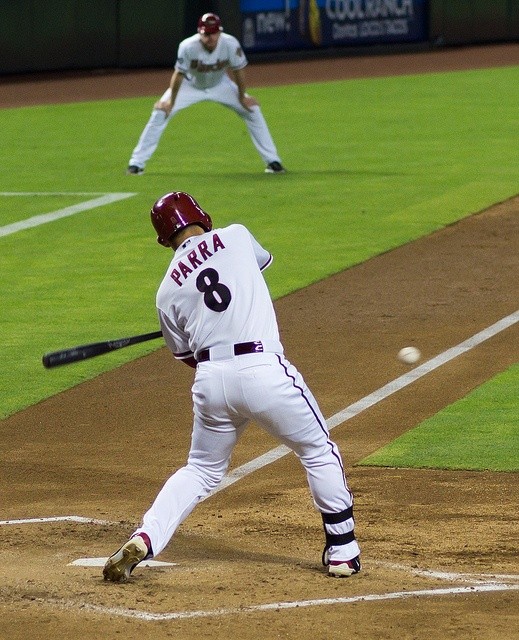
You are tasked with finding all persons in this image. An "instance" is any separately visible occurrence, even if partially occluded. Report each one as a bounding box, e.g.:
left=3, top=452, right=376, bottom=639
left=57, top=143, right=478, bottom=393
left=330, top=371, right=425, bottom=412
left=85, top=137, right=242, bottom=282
left=102, top=190, right=362, bottom=584
left=128, top=10, right=287, bottom=175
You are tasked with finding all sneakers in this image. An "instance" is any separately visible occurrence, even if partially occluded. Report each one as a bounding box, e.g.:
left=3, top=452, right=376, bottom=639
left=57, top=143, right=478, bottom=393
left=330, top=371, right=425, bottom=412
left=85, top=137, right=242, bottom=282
left=329, top=556, right=360, bottom=577
left=265, top=161, right=284, bottom=174
left=103, top=533, right=151, bottom=582
left=127, top=166, right=143, bottom=175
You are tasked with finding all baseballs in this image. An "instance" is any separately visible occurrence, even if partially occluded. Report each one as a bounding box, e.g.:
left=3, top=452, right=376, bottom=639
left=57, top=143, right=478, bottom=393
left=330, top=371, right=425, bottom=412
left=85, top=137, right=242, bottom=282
left=398, top=347, right=419, bottom=363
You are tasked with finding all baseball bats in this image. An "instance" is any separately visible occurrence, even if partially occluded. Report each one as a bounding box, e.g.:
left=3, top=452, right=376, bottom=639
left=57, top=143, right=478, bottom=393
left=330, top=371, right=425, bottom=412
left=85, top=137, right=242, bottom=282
left=42, top=331, right=163, bottom=366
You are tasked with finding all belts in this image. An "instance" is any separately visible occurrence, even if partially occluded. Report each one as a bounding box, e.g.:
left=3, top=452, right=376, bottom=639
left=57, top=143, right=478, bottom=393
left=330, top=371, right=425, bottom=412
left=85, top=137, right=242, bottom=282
left=198, top=341, right=263, bottom=361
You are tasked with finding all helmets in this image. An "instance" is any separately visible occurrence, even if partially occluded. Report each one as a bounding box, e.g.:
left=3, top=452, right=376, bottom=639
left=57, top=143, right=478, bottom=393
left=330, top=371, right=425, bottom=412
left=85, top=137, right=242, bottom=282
left=150, top=192, right=212, bottom=247
left=198, top=13, right=223, bottom=34
